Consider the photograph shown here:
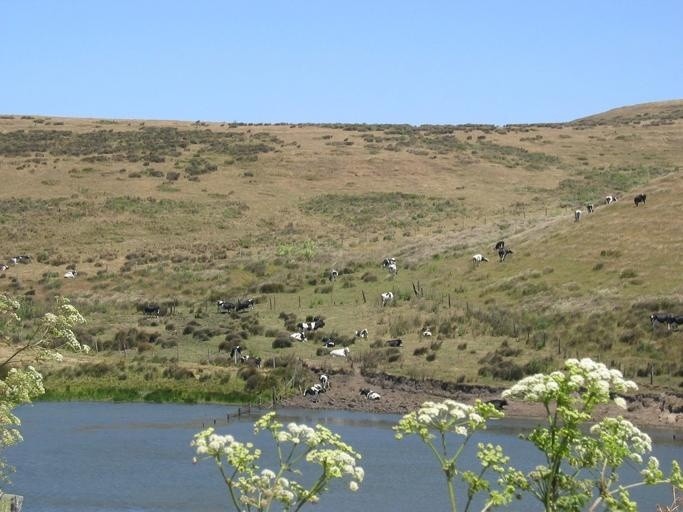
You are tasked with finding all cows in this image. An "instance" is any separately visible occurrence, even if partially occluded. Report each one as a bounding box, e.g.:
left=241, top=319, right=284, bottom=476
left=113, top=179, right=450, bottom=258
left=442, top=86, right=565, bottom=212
left=328, top=270, right=338, bottom=280
left=421, top=327, right=431, bottom=337
left=143, top=305, right=161, bottom=316
left=300, top=372, right=331, bottom=403
left=604, top=194, right=615, bottom=206
left=63, top=269, right=77, bottom=279
left=321, top=336, right=350, bottom=357
left=633, top=193, right=646, bottom=207
left=383, top=257, right=397, bottom=275
left=586, top=202, right=596, bottom=214
left=386, top=337, right=404, bottom=347
left=472, top=254, right=489, bottom=265
left=494, top=240, right=513, bottom=263
left=484, top=398, right=509, bottom=410
left=648, top=310, right=682, bottom=330
left=217, top=298, right=254, bottom=312
left=289, top=319, right=325, bottom=342
left=355, top=328, right=368, bottom=338
left=1, top=254, right=30, bottom=272
left=360, top=387, right=381, bottom=400
left=575, top=209, right=582, bottom=219
left=229, top=344, right=261, bottom=367
left=381, top=291, right=394, bottom=307
